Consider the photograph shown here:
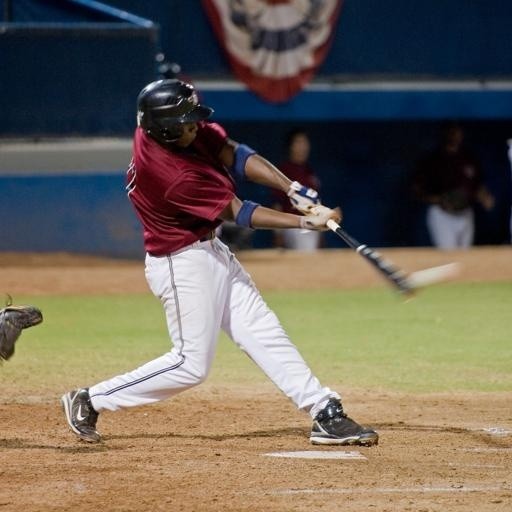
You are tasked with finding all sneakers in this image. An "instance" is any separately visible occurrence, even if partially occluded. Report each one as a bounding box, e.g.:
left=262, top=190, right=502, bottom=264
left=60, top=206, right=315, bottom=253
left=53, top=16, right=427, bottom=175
left=307, top=397, right=379, bottom=445
left=60, top=385, right=102, bottom=443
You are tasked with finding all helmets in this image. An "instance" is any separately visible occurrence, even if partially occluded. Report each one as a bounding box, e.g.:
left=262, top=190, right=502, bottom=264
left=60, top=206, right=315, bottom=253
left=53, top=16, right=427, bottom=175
left=138, top=78, right=215, bottom=143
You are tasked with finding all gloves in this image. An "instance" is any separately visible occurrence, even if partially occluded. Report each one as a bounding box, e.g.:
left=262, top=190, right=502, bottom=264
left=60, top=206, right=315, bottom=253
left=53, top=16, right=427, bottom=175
left=288, top=180, right=341, bottom=233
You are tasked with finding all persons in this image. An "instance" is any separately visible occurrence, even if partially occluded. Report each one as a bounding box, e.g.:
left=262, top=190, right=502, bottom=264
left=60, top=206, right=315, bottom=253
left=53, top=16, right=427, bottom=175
left=420, top=123, right=497, bottom=249
left=62, top=80, right=381, bottom=446
left=270, top=129, right=334, bottom=249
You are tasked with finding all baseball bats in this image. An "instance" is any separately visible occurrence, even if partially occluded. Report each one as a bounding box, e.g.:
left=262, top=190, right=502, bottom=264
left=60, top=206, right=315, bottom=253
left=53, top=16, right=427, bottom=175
left=310, top=205, right=419, bottom=297
